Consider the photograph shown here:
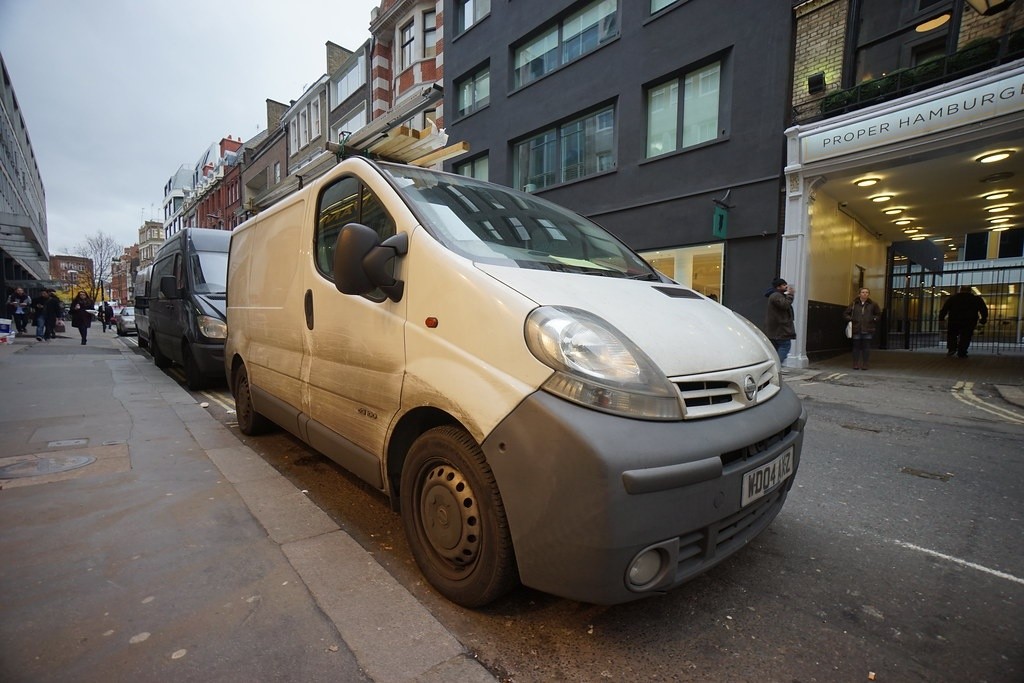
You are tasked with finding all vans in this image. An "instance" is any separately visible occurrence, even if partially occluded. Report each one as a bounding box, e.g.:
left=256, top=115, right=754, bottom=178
left=220, top=158, right=806, bottom=618
left=134, top=263, right=152, bottom=355
left=147, top=227, right=236, bottom=387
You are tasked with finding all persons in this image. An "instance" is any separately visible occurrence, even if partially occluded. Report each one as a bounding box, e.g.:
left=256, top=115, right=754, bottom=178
left=68, top=290, right=94, bottom=345
left=32, top=287, right=65, bottom=342
left=939, top=285, right=988, bottom=361
left=842, top=288, right=884, bottom=370
left=97, top=301, right=114, bottom=332
left=762, top=276, right=797, bottom=362
left=5, top=286, right=32, bottom=335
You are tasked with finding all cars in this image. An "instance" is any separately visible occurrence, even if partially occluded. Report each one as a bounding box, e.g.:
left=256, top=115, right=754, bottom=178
left=110, top=304, right=123, bottom=324
left=115, top=306, right=136, bottom=336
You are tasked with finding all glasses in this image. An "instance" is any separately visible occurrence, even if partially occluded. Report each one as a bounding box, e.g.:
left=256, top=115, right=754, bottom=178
left=79, top=294, right=86, bottom=296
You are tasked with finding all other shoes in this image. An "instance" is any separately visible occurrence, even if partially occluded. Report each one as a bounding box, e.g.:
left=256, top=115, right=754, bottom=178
left=17, top=332, right=22, bottom=337
left=852, top=364, right=868, bottom=370
left=955, top=355, right=969, bottom=361
left=22, top=326, right=27, bottom=333
left=81, top=337, right=87, bottom=345
left=109, top=325, right=111, bottom=329
left=945, top=348, right=957, bottom=356
left=36, top=336, right=43, bottom=342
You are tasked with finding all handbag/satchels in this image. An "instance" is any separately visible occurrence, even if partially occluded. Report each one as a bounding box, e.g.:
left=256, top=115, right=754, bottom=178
left=844, top=300, right=855, bottom=339
left=54, top=318, right=65, bottom=332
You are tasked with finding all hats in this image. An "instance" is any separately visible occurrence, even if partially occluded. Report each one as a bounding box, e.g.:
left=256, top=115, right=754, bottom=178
left=960, top=285, right=973, bottom=292
left=771, top=278, right=786, bottom=288
left=104, top=302, right=109, bottom=306
